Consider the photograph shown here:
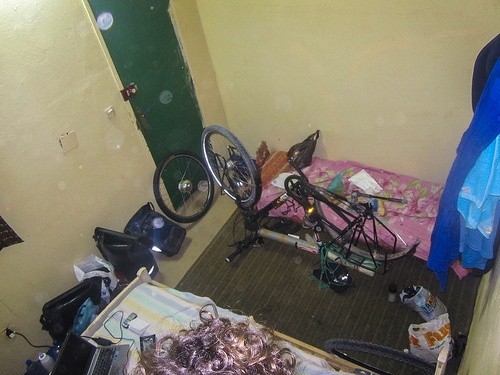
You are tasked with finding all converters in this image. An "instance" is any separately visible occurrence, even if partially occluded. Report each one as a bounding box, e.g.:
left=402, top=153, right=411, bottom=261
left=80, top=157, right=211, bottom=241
left=97, top=338, right=111, bottom=346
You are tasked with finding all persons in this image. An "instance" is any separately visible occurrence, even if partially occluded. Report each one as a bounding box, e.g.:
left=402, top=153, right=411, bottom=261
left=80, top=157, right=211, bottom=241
left=129, top=303, right=297, bottom=375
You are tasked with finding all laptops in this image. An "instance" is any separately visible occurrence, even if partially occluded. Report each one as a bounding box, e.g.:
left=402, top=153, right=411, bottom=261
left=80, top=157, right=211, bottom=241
left=50, top=331, right=128, bottom=375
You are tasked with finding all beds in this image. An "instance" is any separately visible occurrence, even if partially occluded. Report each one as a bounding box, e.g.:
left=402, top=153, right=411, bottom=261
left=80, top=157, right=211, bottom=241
left=240, top=151, right=472, bottom=280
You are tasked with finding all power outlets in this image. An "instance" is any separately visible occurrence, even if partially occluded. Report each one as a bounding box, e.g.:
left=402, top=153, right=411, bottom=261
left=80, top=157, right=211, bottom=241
left=1, top=327, right=10, bottom=338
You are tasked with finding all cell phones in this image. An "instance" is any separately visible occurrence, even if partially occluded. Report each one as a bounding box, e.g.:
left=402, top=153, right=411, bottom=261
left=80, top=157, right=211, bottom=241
left=122, top=312, right=137, bottom=328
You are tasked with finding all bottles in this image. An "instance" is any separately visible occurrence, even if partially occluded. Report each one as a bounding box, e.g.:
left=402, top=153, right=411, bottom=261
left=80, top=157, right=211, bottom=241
left=38, top=351, right=55, bottom=372
left=26, top=359, right=40, bottom=375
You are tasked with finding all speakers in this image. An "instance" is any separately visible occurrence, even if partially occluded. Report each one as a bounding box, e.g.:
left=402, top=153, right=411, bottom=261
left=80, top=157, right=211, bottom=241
left=94, top=227, right=159, bottom=282
left=125, top=204, right=186, bottom=257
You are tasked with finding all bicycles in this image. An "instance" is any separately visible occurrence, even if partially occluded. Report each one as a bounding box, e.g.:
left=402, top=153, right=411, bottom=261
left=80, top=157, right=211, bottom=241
left=200, top=124, right=421, bottom=295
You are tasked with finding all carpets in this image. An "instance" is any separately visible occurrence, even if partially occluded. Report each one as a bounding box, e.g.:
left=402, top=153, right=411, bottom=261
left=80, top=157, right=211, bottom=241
left=174, top=206, right=481, bottom=375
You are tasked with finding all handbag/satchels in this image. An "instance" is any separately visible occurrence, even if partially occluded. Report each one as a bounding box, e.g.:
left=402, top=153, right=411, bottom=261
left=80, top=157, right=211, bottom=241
left=73, top=254, right=120, bottom=293
left=400, top=286, right=448, bottom=320
left=407, top=312, right=453, bottom=366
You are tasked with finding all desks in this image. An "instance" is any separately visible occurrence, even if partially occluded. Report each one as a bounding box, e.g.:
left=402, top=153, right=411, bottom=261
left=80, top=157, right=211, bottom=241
left=79, top=268, right=379, bottom=375
